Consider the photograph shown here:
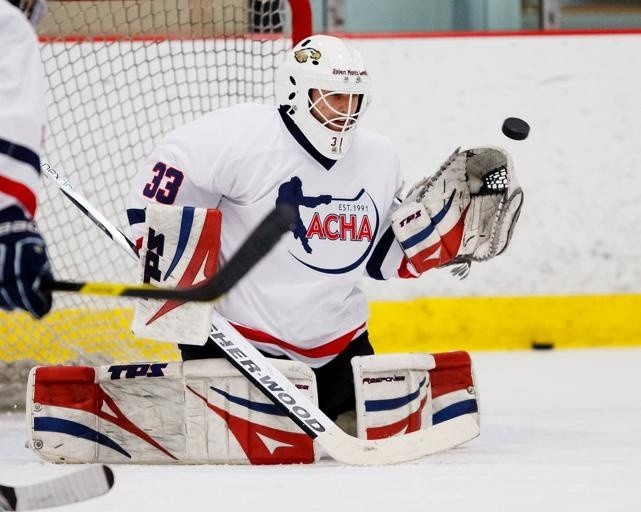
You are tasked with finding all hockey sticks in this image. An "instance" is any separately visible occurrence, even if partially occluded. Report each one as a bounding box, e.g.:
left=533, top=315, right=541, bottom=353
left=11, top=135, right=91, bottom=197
left=37, top=158, right=479, bottom=465
left=55, top=202, right=296, bottom=302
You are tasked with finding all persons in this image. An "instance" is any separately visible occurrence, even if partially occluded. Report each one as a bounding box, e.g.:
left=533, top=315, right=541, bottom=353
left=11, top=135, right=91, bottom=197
left=0, top=0, right=54, bottom=319
left=125, top=34, right=525, bottom=422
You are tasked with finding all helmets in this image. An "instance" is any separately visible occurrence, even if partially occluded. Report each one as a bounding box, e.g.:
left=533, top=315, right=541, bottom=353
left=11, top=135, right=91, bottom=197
left=277, top=35, right=369, bottom=160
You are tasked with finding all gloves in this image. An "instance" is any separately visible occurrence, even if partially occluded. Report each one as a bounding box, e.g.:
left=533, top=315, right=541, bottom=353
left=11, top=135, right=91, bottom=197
left=0, top=205, right=51, bottom=318
